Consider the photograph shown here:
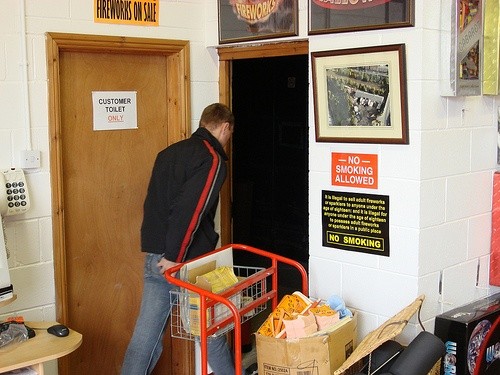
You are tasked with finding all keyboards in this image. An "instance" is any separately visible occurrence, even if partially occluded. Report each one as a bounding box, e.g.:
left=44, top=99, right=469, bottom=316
left=0, top=321, right=35, bottom=339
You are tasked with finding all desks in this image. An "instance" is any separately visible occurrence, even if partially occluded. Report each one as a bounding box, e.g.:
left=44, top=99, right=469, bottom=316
left=0, top=320, right=84, bottom=375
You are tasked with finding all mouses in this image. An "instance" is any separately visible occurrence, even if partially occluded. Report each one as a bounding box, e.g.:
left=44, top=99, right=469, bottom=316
left=47, top=324, right=69, bottom=337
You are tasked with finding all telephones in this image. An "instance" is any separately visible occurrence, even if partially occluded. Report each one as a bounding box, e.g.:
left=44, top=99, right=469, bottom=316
left=0, top=168, right=31, bottom=259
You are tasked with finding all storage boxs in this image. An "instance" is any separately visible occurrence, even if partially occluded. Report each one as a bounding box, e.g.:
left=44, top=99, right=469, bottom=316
left=255, top=305, right=359, bottom=375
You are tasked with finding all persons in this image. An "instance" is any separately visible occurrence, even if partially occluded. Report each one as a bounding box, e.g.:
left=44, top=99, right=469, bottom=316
left=119, top=103, right=245, bottom=375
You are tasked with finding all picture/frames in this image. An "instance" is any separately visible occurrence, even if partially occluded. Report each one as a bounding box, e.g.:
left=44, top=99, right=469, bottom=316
left=311, top=44, right=410, bottom=145
left=218, top=0, right=298, bottom=44
left=308, top=0, right=415, bottom=35
